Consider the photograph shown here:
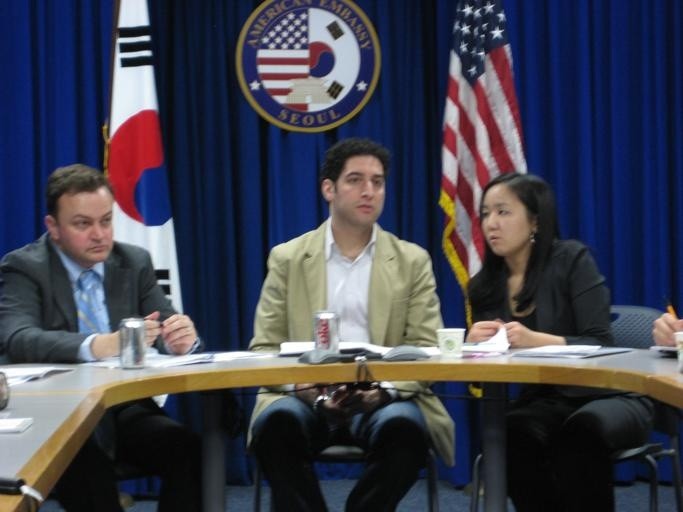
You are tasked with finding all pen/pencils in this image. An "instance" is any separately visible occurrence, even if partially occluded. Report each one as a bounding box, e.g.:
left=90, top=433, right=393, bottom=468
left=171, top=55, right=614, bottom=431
left=662, top=296, right=679, bottom=320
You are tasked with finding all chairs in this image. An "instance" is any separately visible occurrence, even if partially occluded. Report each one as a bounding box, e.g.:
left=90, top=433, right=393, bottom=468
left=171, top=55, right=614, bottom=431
left=470, top=304, right=682, bottom=511
left=252, top=435, right=439, bottom=512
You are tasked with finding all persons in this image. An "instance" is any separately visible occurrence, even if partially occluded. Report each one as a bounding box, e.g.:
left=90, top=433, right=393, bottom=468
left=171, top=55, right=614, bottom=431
left=0, top=163, right=205, bottom=512
left=246, top=135, right=456, bottom=511
left=466, top=172, right=612, bottom=511
left=557, top=312, right=682, bottom=511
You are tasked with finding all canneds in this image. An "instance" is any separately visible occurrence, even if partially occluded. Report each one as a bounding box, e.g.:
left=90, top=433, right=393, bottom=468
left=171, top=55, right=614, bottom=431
left=119, top=319, right=148, bottom=369
left=314, top=311, right=339, bottom=351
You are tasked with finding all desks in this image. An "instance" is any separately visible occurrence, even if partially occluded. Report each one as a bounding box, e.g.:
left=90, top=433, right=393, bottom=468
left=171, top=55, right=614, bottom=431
left=1, top=343, right=683, bottom=512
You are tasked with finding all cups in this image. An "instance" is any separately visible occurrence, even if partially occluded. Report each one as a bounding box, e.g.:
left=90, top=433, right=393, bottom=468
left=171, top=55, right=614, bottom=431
left=436, top=328, right=465, bottom=359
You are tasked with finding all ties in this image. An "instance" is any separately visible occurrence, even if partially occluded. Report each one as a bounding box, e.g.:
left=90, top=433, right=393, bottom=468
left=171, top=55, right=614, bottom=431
left=75, top=272, right=116, bottom=459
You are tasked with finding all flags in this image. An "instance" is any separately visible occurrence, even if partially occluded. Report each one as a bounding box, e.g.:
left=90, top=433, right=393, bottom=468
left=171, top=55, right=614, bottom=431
left=100, top=0, right=186, bottom=410
left=439, top=0, right=528, bottom=399
